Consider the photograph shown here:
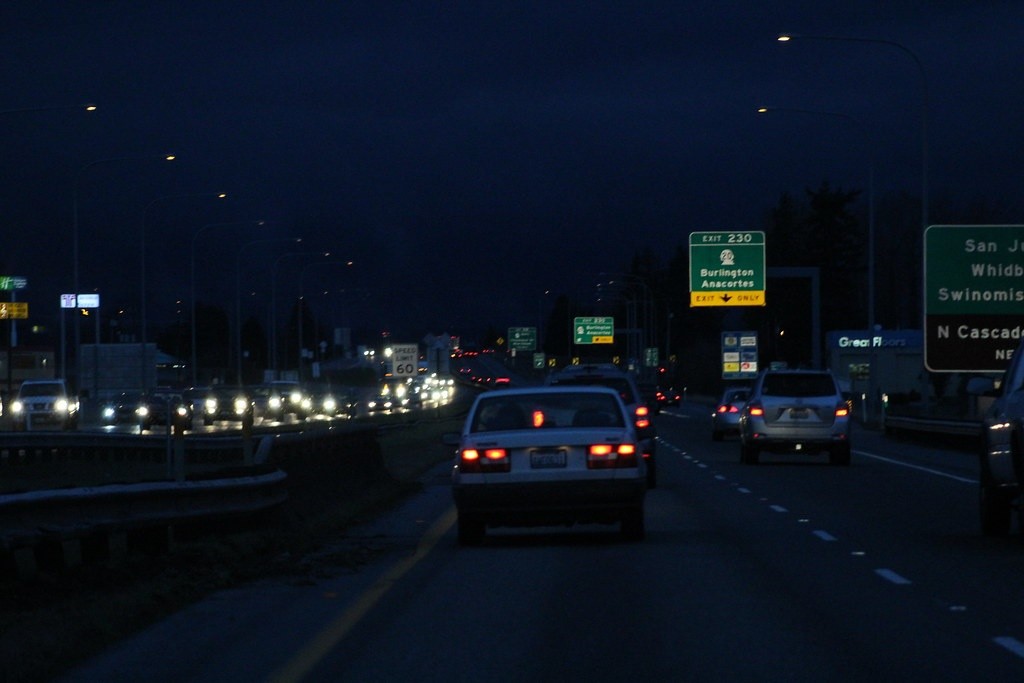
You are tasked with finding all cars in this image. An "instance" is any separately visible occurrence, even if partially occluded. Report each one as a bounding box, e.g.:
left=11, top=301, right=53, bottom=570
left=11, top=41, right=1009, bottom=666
left=550, top=367, right=658, bottom=472
left=648, top=372, right=685, bottom=405
left=12, top=378, right=83, bottom=429
left=99, top=391, right=152, bottom=427
left=442, top=385, right=654, bottom=539
left=203, top=386, right=258, bottom=431
left=264, top=374, right=451, bottom=421
left=149, top=392, right=193, bottom=428
left=963, top=335, right=1024, bottom=508
left=712, top=387, right=753, bottom=439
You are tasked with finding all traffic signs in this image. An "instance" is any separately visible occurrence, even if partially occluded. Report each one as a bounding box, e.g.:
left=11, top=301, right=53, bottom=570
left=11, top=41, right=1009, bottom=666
left=689, top=232, right=769, bottom=306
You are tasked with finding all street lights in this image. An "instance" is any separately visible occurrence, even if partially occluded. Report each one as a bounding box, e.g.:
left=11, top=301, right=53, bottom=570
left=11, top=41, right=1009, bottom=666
left=142, top=190, right=228, bottom=385
left=270, top=250, right=332, bottom=370
left=191, top=218, right=267, bottom=382
left=295, top=258, right=355, bottom=370
left=72, top=154, right=177, bottom=374
left=61, top=103, right=100, bottom=380
left=758, top=102, right=876, bottom=418
left=778, top=32, right=930, bottom=227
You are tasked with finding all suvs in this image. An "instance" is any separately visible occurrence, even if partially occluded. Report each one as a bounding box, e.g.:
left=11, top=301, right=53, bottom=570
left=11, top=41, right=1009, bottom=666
left=735, top=364, right=855, bottom=466
